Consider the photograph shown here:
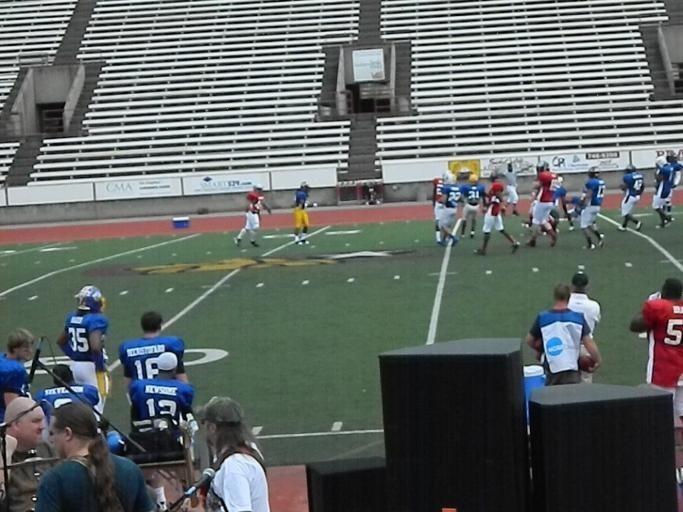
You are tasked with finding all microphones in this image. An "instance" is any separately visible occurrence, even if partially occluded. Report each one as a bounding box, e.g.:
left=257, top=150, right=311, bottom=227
left=186, top=466, right=218, bottom=496
left=23, top=336, right=45, bottom=391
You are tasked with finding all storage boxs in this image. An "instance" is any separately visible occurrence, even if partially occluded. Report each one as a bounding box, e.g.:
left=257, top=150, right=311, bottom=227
left=172, top=216, right=191, bottom=228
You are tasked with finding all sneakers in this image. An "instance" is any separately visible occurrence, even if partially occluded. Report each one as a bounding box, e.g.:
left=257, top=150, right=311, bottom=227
left=233, top=237, right=259, bottom=247
left=436, top=230, right=474, bottom=247
left=582, top=234, right=605, bottom=250
left=512, top=242, right=520, bottom=254
left=616, top=216, right=642, bottom=232
left=656, top=215, right=674, bottom=229
left=294, top=239, right=309, bottom=246
left=473, top=249, right=486, bottom=255
left=522, top=222, right=575, bottom=246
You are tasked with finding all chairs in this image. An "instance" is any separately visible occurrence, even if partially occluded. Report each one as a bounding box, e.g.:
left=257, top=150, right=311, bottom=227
left=124, top=428, right=199, bottom=506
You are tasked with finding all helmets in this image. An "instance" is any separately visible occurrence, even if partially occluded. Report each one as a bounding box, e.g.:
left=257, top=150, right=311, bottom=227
left=536, top=160, right=550, bottom=170
left=300, top=182, right=308, bottom=190
left=588, top=166, right=600, bottom=177
left=445, top=173, right=458, bottom=185
left=625, top=164, right=635, bottom=172
left=254, top=183, right=262, bottom=191
left=74, top=286, right=104, bottom=313
left=667, top=150, right=678, bottom=162
left=469, top=174, right=478, bottom=184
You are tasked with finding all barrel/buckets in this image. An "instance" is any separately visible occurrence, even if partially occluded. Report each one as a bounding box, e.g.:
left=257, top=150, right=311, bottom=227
left=523, top=365, right=546, bottom=425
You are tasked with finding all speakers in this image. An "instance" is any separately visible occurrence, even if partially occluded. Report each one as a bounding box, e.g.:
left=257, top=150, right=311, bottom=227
left=304, top=455, right=442, bottom=512
left=528, top=383, right=678, bottom=512
left=377, top=337, right=529, bottom=508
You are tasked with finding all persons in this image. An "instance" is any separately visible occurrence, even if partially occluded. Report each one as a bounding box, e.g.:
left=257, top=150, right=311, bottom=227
left=33, top=365, right=100, bottom=423
left=1, top=329, right=35, bottom=419
left=525, top=282, right=602, bottom=386
left=1, top=397, right=160, bottom=510
left=567, top=271, right=602, bottom=383
left=433, top=154, right=683, bottom=255
left=57, top=286, right=110, bottom=415
left=293, top=181, right=311, bottom=245
left=629, top=278, right=683, bottom=484
left=194, top=396, right=271, bottom=511
left=233, top=184, right=272, bottom=247
left=119, top=312, right=198, bottom=444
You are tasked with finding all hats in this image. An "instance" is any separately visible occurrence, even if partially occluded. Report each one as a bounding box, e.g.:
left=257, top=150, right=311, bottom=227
left=158, top=353, right=179, bottom=370
left=194, top=396, right=245, bottom=425
